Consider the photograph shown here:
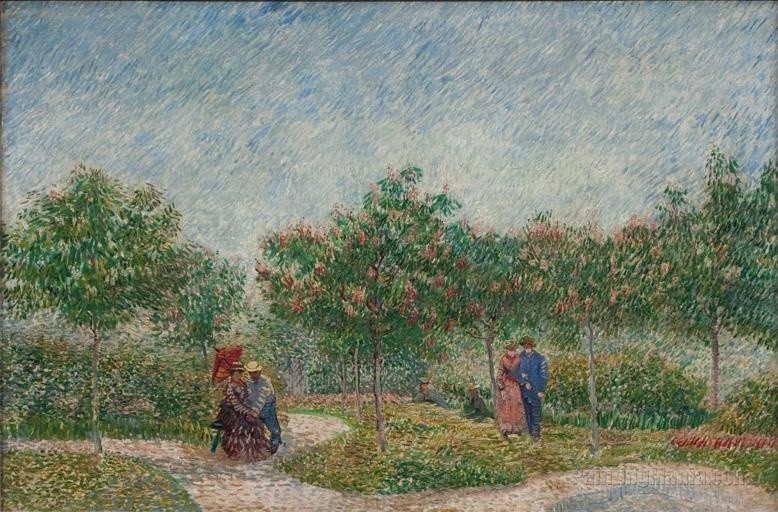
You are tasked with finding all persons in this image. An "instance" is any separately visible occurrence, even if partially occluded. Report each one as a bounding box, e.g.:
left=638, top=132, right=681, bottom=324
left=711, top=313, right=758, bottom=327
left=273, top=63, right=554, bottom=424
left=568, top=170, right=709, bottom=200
left=508, top=335, right=549, bottom=442
left=240, top=361, right=282, bottom=455
left=209, top=362, right=244, bottom=453
left=495, top=342, right=525, bottom=438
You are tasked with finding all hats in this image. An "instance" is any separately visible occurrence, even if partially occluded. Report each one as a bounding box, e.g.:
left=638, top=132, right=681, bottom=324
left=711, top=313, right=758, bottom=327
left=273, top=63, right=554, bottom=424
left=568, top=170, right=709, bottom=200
left=244, top=361, right=262, bottom=372
left=519, top=336, right=536, bottom=347
left=229, top=362, right=245, bottom=373
left=503, top=340, right=518, bottom=351
left=465, top=383, right=479, bottom=391
left=418, top=379, right=429, bottom=383
